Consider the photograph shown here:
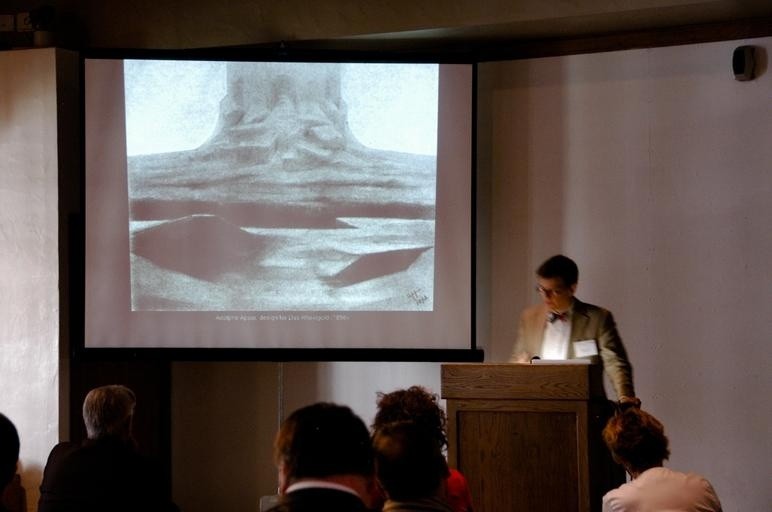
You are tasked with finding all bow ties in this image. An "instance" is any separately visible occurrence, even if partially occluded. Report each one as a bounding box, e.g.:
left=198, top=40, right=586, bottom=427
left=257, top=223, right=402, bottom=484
left=545, top=311, right=569, bottom=323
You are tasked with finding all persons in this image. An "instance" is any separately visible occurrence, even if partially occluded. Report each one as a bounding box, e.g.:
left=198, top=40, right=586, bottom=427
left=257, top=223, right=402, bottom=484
left=601, top=408, right=723, bottom=512
left=371, top=385, right=473, bottom=511
left=375, top=440, right=462, bottom=512
left=507, top=254, right=641, bottom=406
left=256, top=401, right=380, bottom=512
left=0, top=413, right=27, bottom=512
left=37, top=386, right=173, bottom=511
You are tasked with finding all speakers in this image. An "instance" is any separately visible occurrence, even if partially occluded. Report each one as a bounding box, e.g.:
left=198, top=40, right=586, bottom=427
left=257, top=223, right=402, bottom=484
left=731, top=45, right=759, bottom=81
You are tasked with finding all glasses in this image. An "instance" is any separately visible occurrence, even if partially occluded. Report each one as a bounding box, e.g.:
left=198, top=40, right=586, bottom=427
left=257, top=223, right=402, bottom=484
left=535, top=286, right=567, bottom=298
